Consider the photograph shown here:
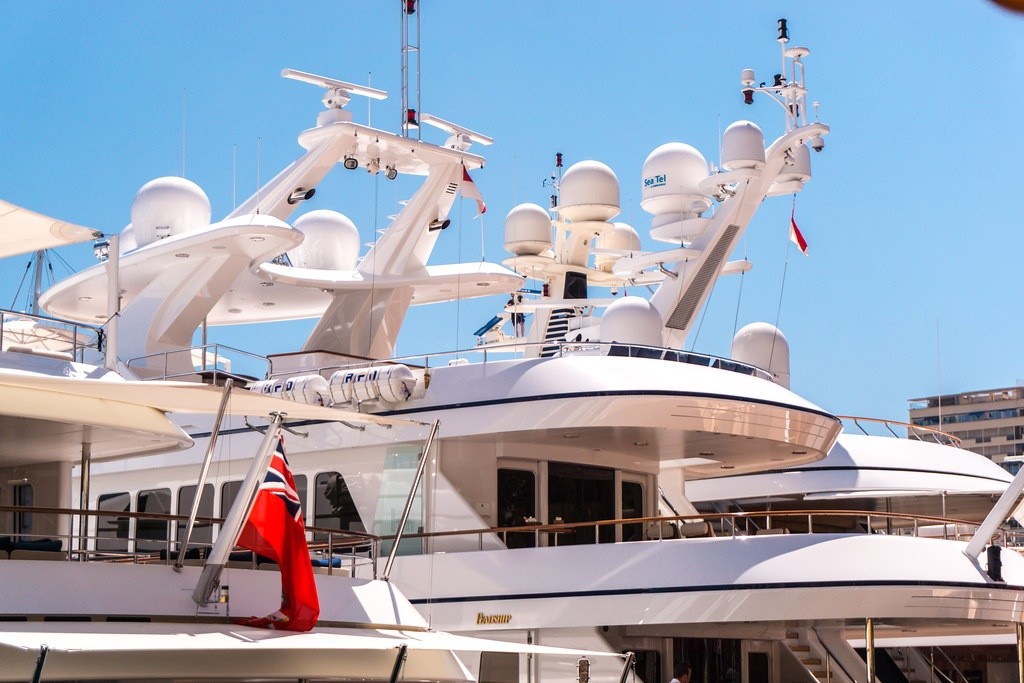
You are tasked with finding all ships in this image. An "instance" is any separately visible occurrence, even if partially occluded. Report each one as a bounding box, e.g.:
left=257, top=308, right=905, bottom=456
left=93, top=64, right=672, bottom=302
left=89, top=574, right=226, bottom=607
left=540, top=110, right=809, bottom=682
left=0, top=2, right=1024, bottom=683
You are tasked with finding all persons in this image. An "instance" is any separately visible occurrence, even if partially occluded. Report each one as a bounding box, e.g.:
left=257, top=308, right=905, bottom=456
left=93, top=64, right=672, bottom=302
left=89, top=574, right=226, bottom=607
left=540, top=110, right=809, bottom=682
left=504, top=292, right=526, bottom=338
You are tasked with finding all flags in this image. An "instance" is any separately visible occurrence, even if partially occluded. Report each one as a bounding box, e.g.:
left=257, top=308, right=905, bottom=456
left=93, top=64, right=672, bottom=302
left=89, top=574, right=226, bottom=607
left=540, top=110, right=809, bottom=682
left=461, top=162, right=487, bottom=215
left=233, top=436, right=322, bottom=633
left=789, top=218, right=807, bottom=254
